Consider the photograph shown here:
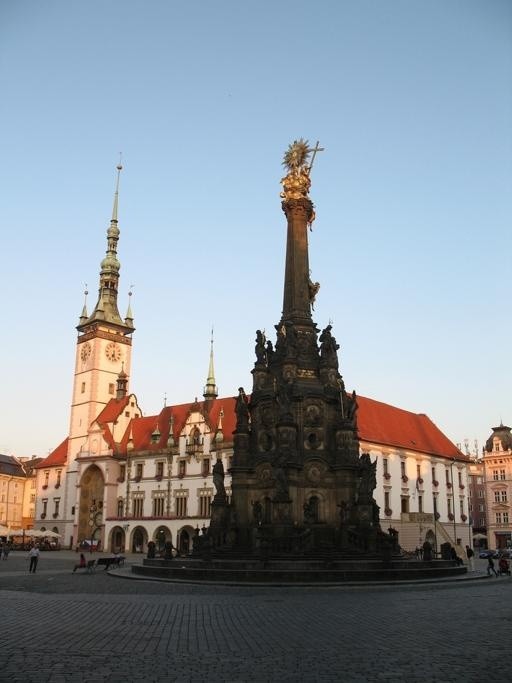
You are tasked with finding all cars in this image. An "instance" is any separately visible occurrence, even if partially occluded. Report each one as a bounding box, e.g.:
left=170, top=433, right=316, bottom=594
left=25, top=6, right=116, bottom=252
left=479, top=546, right=512, bottom=558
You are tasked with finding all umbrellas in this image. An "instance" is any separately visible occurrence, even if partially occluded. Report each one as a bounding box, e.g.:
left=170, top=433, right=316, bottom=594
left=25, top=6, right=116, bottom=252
left=473, top=533, right=487, bottom=552
left=9, top=529, right=62, bottom=538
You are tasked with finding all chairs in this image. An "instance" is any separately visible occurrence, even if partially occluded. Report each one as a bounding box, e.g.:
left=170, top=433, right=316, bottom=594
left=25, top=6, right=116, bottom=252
left=79, top=557, right=127, bottom=574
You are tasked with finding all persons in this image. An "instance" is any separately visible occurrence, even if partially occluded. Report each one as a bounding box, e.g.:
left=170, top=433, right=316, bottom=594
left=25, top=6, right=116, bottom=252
left=0, top=540, right=11, bottom=560
left=72, top=553, right=86, bottom=572
left=486, top=553, right=498, bottom=578
left=212, top=325, right=379, bottom=505
left=28, top=539, right=57, bottom=551
left=29, top=544, right=40, bottom=573
left=465, top=545, right=475, bottom=572
